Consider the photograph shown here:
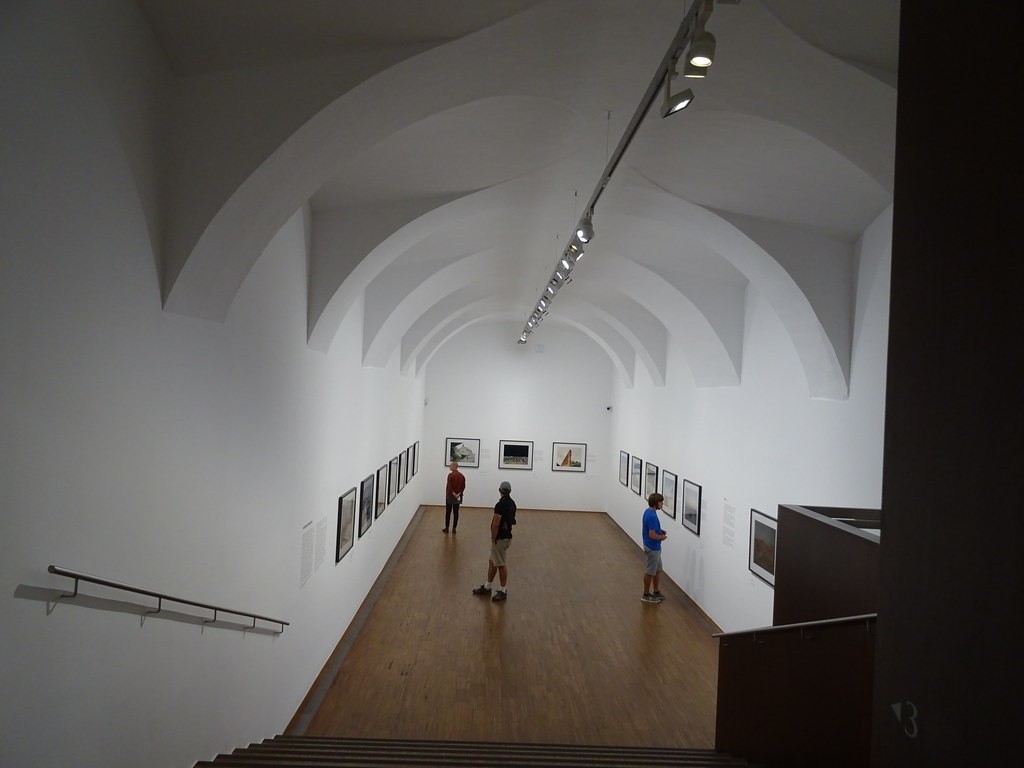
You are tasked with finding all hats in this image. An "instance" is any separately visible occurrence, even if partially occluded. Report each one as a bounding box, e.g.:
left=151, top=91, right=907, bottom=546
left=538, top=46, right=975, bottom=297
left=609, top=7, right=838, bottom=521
left=500, top=481, right=511, bottom=493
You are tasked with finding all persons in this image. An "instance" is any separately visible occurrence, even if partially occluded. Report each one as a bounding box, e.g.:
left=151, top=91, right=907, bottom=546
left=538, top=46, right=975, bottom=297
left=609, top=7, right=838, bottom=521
left=442, top=462, right=465, bottom=534
left=640, top=493, right=668, bottom=603
left=472, top=481, right=517, bottom=601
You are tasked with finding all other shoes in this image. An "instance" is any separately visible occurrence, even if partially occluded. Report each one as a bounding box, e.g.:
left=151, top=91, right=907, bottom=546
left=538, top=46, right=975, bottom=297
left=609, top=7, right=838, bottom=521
left=492, top=589, right=507, bottom=601
left=473, top=585, right=491, bottom=596
left=452, top=528, right=457, bottom=534
left=442, top=528, right=449, bottom=533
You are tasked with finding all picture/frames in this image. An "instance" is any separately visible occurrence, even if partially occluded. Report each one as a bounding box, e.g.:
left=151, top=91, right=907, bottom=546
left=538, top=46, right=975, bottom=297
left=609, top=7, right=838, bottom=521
left=375, top=464, right=387, bottom=519
left=358, top=474, right=374, bottom=538
left=748, top=509, right=777, bottom=588
left=682, top=479, right=701, bottom=535
left=336, top=487, right=357, bottom=563
left=644, top=462, right=658, bottom=501
left=619, top=451, right=629, bottom=486
left=552, top=442, right=587, bottom=472
left=499, top=440, right=533, bottom=470
left=387, top=457, right=397, bottom=504
left=660, top=470, right=677, bottom=519
left=445, top=437, right=480, bottom=468
left=630, top=456, right=642, bottom=495
left=398, top=441, right=419, bottom=493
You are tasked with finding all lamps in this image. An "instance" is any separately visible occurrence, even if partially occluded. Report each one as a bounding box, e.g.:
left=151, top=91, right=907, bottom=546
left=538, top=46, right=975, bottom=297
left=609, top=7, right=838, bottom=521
left=521, top=208, right=594, bottom=342
left=683, top=11, right=715, bottom=80
left=659, top=57, right=695, bottom=118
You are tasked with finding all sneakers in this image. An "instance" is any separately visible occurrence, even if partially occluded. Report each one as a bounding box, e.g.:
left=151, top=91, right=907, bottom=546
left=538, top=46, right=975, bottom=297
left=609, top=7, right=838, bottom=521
left=641, top=593, right=660, bottom=603
left=653, top=592, right=664, bottom=598
